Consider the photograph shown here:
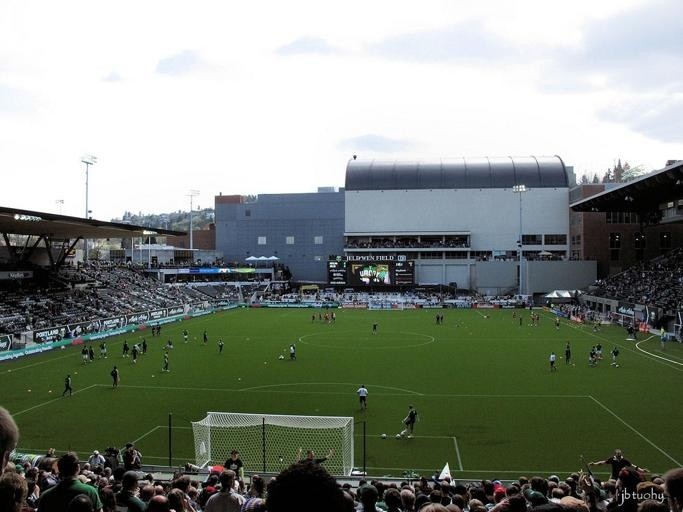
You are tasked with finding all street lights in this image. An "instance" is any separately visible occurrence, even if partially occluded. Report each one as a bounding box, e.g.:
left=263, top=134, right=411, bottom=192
left=184, top=188, right=200, bottom=249
left=512, top=185, right=526, bottom=294
left=79, top=153, right=98, bottom=270
left=88, top=209, right=94, bottom=248
left=54, top=199, right=64, bottom=215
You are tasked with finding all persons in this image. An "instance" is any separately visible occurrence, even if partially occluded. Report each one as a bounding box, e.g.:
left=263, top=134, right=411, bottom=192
left=61, top=372, right=73, bottom=396
left=440, top=313, right=444, bottom=327
left=434, top=313, right=440, bottom=327
left=288, top=342, right=297, bottom=360
left=310, top=311, right=336, bottom=324
left=160, top=352, right=169, bottom=372
left=110, top=366, right=120, bottom=388
left=356, top=384, right=369, bottom=410
left=371, top=320, right=377, bottom=334
left=0, top=254, right=279, bottom=364
left=0, top=406, right=683, bottom=512
left=479, top=236, right=682, bottom=371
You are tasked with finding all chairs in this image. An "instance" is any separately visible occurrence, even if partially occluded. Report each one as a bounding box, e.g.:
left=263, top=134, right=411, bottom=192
left=0, top=260, right=232, bottom=333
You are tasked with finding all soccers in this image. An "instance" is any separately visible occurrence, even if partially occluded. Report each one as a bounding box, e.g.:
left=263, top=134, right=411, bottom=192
left=278, top=353, right=285, bottom=360
left=615, top=364, right=619, bottom=368
left=379, top=429, right=408, bottom=440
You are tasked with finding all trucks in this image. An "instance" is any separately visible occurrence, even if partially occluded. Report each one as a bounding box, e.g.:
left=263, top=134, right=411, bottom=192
left=511, top=294, right=532, bottom=306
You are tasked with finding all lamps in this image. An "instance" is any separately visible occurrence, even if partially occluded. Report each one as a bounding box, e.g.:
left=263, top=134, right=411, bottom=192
left=13, top=212, right=41, bottom=223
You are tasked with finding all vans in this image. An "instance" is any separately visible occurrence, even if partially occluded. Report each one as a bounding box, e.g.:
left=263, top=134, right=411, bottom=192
left=320, top=287, right=355, bottom=293
